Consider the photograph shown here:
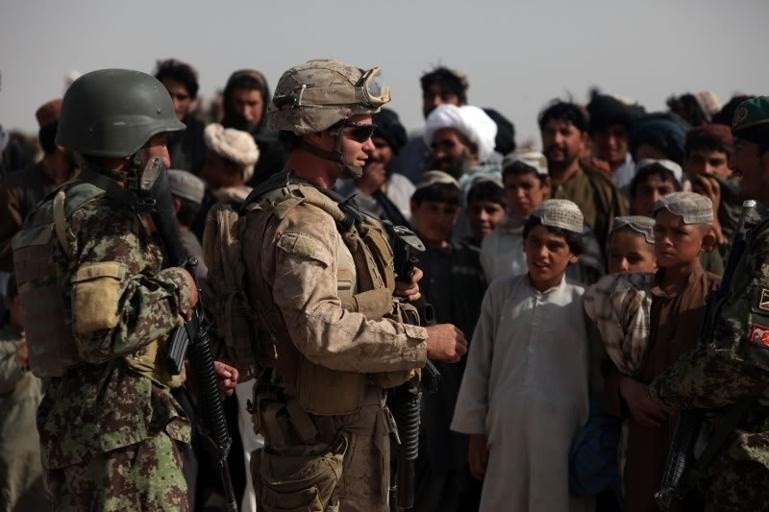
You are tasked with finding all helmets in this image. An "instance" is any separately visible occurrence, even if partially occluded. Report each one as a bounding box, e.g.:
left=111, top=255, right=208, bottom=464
left=265, top=59, right=382, bottom=136
left=54, top=68, right=188, bottom=158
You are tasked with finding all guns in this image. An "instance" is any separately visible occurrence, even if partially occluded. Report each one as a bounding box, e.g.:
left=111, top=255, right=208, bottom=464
left=393, top=224, right=441, bottom=512
left=653, top=201, right=758, bottom=511
left=139, top=155, right=240, bottom=511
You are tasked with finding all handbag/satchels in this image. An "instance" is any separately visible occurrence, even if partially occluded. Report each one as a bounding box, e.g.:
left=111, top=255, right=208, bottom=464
left=258, top=453, right=347, bottom=512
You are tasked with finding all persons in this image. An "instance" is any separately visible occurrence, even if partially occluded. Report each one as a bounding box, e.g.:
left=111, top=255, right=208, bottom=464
left=2, top=54, right=769, bottom=512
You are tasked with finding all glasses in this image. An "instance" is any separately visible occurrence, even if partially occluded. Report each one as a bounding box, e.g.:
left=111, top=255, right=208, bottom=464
left=343, top=119, right=376, bottom=143
left=354, top=66, right=393, bottom=109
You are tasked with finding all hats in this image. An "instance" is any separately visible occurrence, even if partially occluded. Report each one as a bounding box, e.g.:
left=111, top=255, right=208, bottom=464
left=35, top=98, right=64, bottom=128
left=501, top=151, right=548, bottom=175
left=692, top=88, right=724, bottom=123
left=654, top=192, right=715, bottom=225
left=469, top=172, right=504, bottom=189
left=530, top=197, right=583, bottom=234
left=685, top=123, right=732, bottom=146
left=730, top=95, right=768, bottom=135
left=633, top=158, right=683, bottom=188
left=612, top=215, right=658, bottom=245
left=415, top=170, right=461, bottom=192
left=164, top=167, right=206, bottom=206
